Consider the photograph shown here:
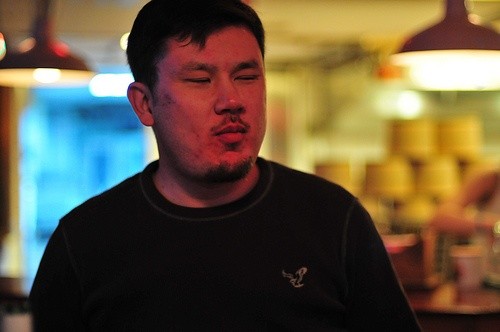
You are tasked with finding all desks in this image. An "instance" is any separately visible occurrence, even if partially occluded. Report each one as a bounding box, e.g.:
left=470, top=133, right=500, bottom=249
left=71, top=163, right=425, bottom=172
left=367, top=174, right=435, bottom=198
left=411, top=287, right=500, bottom=332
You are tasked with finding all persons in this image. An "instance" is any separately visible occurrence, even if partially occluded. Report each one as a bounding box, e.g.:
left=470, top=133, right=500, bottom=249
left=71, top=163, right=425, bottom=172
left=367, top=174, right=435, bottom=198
left=30, top=0, right=422, bottom=332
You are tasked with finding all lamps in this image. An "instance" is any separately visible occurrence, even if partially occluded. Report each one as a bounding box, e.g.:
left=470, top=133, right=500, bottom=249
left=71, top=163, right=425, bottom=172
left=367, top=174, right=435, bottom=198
left=387, top=0, right=500, bottom=70
left=0, top=0, right=95, bottom=87
